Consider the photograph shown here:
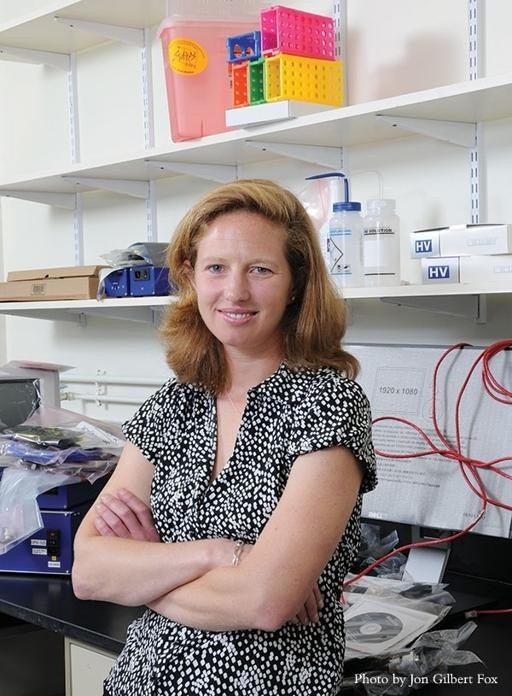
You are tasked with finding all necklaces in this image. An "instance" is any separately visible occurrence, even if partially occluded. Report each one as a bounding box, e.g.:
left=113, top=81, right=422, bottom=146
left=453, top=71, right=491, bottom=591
left=222, top=383, right=243, bottom=420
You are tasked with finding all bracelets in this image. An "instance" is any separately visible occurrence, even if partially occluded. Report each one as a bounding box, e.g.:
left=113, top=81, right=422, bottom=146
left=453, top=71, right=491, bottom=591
left=231, top=538, right=245, bottom=568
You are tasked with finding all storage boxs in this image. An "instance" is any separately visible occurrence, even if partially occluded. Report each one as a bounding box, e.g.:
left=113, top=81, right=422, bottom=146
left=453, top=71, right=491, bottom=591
left=0, top=503, right=93, bottom=576
left=410, top=223, right=511, bottom=259
left=0, top=265, right=112, bottom=301
left=420, top=256, right=512, bottom=285
left=158, top=14, right=262, bottom=142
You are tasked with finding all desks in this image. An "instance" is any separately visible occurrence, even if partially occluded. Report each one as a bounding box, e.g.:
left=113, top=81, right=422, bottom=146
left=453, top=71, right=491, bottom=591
left=1, top=514, right=511, bottom=696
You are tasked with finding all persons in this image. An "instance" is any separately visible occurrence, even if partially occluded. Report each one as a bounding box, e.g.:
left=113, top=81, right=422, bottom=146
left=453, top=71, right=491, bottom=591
left=71, top=180, right=379, bottom=696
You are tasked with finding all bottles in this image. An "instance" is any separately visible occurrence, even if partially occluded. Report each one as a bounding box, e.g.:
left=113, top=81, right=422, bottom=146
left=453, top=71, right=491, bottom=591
left=362, top=198, right=401, bottom=288
left=327, top=201, right=362, bottom=288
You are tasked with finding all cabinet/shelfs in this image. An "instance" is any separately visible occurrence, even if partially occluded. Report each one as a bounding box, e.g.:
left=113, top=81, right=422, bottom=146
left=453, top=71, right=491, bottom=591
left=1, top=1, right=511, bottom=326
